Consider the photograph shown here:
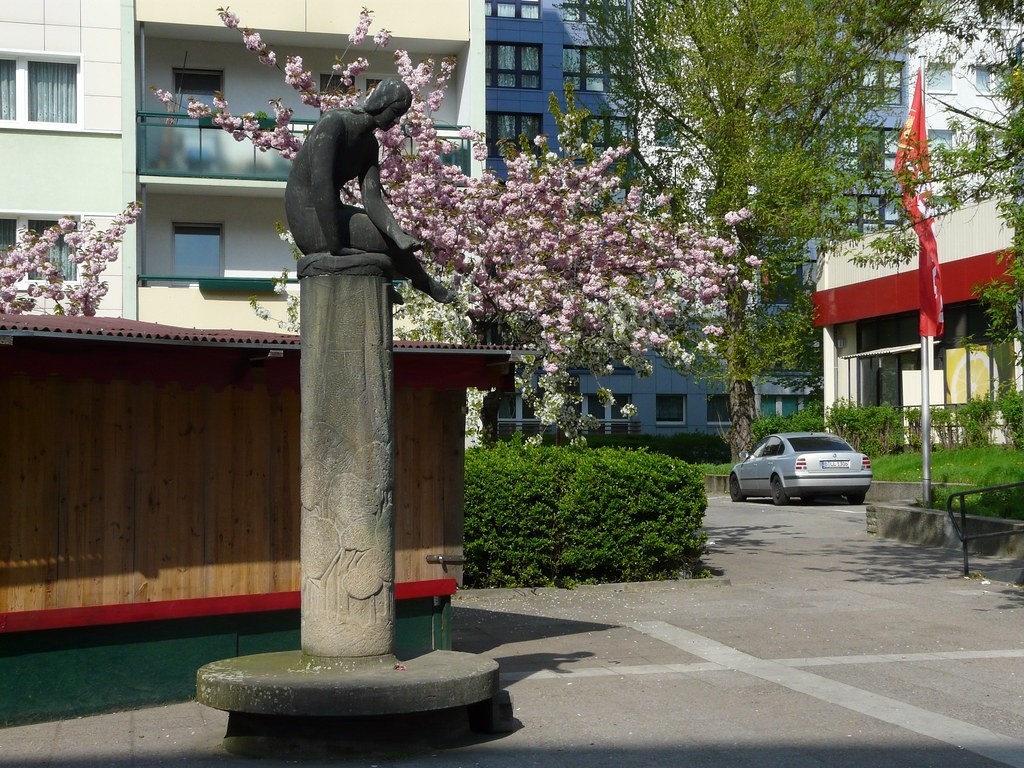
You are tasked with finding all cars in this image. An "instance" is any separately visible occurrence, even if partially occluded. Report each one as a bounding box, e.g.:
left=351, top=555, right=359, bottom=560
left=729, top=431, right=873, bottom=506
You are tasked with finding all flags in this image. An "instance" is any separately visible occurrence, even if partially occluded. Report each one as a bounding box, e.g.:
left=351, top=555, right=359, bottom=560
left=893, top=66, right=946, bottom=340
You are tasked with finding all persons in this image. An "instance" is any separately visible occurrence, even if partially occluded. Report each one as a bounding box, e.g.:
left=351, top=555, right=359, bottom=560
left=284, top=77, right=458, bottom=304
